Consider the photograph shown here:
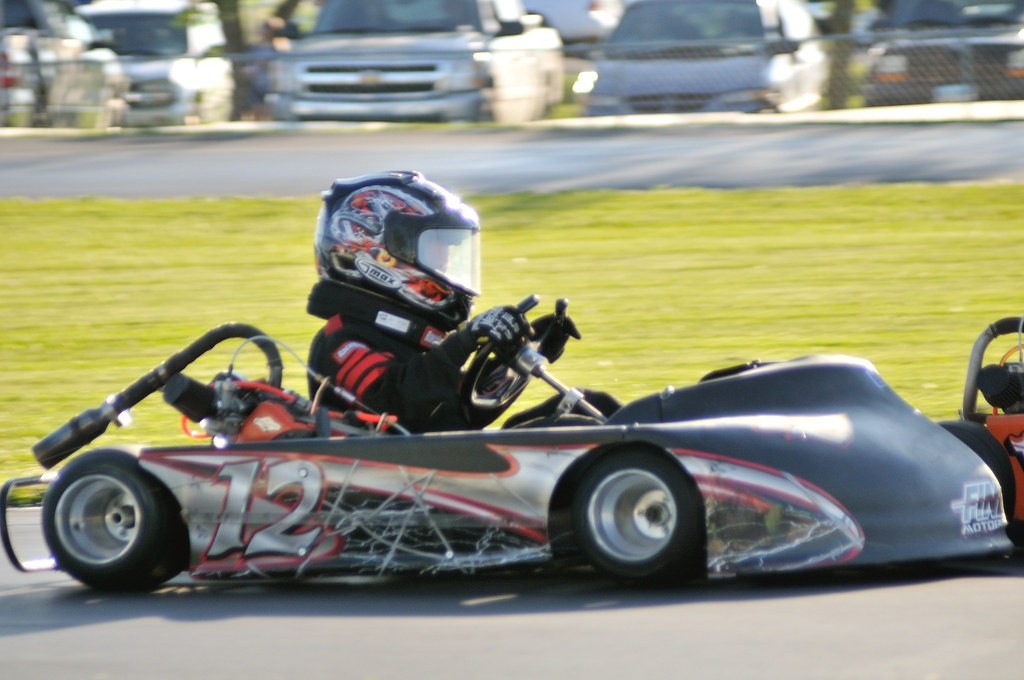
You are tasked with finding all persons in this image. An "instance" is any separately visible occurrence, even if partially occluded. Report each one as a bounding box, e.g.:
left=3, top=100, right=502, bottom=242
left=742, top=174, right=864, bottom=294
left=241, top=14, right=292, bottom=123
left=305, top=167, right=625, bottom=435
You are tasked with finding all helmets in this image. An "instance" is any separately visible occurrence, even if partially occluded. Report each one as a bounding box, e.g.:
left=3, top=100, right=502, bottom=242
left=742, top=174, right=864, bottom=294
left=306, top=171, right=481, bottom=353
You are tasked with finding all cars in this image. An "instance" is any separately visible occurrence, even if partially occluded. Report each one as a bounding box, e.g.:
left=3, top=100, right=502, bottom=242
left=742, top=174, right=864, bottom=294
left=858, top=0, right=1024, bottom=107
left=573, top=1, right=835, bottom=116
left=79, top=1, right=236, bottom=127
left=0, top=0, right=132, bottom=123
left=529, top=1, right=624, bottom=42
left=258, top=1, right=565, bottom=125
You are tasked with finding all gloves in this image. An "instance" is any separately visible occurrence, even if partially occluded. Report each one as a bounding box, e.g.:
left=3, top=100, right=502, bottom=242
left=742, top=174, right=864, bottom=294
left=527, top=313, right=582, bottom=362
left=458, top=305, right=535, bottom=366
